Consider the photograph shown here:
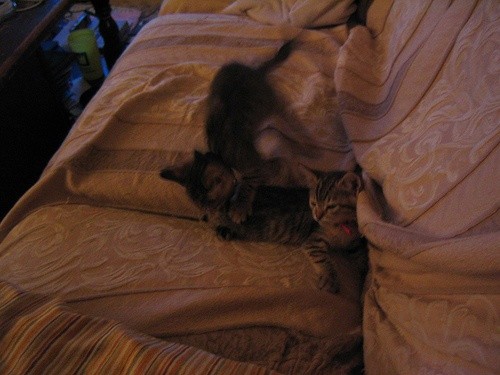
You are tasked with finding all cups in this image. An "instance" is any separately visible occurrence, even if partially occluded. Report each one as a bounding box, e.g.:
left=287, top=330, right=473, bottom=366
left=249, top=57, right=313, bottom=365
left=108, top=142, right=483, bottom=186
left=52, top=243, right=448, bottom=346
left=67, top=28, right=102, bottom=80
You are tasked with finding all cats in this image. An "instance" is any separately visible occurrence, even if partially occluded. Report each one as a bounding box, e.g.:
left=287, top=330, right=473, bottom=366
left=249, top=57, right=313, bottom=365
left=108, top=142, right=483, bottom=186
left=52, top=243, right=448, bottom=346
left=201, top=163, right=361, bottom=294
left=160, top=39, right=306, bottom=224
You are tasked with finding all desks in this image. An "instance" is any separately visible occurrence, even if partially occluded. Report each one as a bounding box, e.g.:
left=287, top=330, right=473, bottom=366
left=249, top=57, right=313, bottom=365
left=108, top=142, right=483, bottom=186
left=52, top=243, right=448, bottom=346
left=0, top=0, right=122, bottom=79
